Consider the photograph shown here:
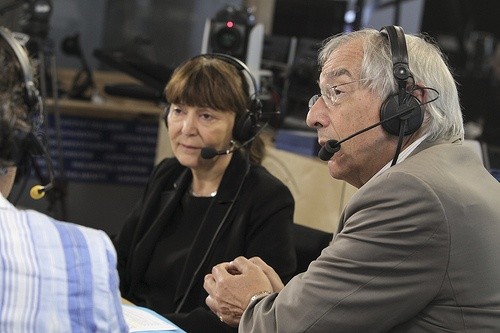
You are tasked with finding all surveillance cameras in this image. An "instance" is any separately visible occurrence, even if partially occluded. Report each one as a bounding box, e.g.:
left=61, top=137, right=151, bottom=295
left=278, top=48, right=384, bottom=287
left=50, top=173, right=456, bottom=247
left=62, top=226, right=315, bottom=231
left=200, top=9, right=264, bottom=99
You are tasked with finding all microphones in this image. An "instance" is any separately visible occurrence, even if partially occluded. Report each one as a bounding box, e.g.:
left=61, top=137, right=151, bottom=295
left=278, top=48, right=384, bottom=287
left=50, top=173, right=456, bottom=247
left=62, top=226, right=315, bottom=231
left=29, top=134, right=55, bottom=199
left=319, top=107, right=415, bottom=161
left=201, top=122, right=269, bottom=160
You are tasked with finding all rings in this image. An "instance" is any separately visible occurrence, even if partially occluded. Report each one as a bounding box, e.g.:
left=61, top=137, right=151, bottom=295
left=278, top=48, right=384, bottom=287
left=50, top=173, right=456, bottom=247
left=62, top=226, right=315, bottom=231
left=219, top=315, right=223, bottom=322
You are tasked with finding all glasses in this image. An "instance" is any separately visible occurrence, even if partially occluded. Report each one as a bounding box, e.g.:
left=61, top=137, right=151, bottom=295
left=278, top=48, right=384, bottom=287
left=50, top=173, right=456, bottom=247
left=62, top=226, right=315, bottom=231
left=307, top=77, right=372, bottom=109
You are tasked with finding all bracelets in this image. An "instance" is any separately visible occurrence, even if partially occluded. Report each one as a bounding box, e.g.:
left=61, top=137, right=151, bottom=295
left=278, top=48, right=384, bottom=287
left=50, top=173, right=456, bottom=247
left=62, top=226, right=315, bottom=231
left=250, top=291, right=272, bottom=303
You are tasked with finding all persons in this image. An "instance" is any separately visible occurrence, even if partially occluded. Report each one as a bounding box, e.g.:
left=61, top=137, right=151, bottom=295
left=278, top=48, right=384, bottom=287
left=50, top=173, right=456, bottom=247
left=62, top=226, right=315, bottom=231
left=203, top=27, right=500, bottom=333
left=0, top=32, right=130, bottom=333
left=111, top=54, right=298, bottom=333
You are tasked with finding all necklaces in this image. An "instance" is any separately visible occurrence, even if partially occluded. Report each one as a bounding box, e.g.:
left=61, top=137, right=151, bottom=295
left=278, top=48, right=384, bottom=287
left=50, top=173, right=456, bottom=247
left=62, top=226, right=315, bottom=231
left=189, top=187, right=217, bottom=197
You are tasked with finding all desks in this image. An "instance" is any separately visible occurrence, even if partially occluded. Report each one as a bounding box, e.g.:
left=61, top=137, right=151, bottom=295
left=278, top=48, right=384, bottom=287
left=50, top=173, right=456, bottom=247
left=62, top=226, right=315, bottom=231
left=41, top=68, right=167, bottom=183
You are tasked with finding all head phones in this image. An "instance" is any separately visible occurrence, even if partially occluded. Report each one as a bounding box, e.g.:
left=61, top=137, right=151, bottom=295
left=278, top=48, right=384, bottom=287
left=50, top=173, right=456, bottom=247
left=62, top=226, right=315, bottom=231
left=379, top=25, right=425, bottom=136
left=162, top=54, right=265, bottom=143
left=0, top=25, right=43, bottom=166
